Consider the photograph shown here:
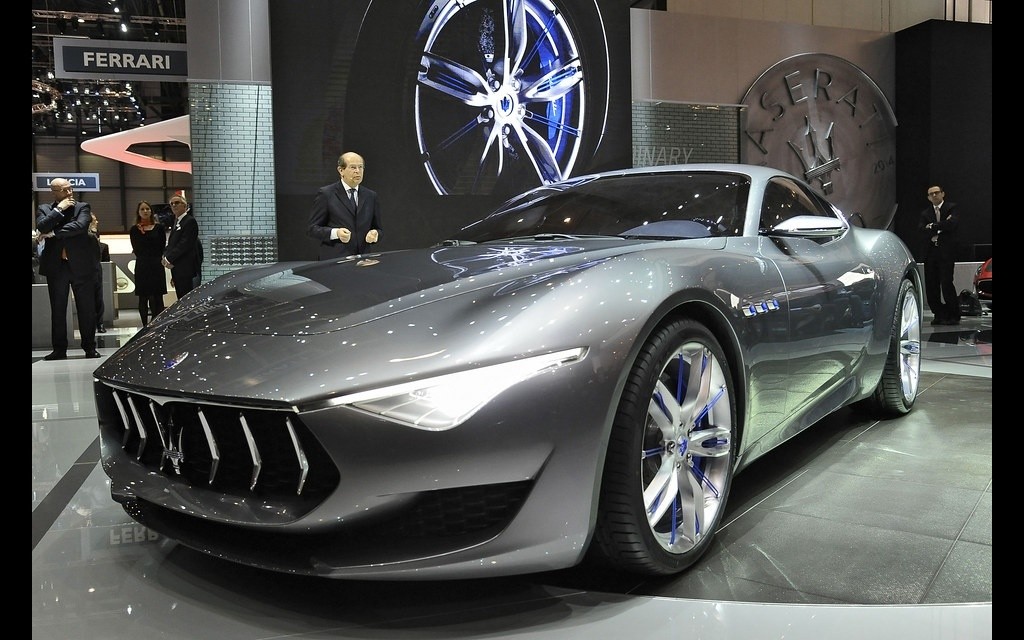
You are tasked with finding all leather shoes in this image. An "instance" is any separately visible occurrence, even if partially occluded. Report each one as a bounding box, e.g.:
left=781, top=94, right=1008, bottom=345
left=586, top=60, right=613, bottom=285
left=44, top=350, right=66, bottom=360
left=85, top=350, right=101, bottom=358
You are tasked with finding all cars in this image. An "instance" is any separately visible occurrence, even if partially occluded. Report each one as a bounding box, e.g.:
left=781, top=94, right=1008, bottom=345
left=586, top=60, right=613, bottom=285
left=970, top=258, right=992, bottom=303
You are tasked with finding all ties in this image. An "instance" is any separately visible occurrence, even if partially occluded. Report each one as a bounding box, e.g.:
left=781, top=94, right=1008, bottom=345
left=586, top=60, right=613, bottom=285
left=349, top=188, right=357, bottom=213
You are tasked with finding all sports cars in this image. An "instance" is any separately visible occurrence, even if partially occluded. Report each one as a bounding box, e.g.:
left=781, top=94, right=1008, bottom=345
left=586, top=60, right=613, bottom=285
left=88, top=163, right=926, bottom=582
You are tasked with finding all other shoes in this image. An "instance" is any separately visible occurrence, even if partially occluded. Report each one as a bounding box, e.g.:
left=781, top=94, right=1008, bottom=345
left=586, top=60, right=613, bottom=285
left=98, top=324, right=106, bottom=333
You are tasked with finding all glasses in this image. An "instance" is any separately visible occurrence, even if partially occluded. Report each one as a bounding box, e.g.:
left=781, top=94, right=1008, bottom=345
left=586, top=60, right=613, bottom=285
left=341, top=165, right=365, bottom=171
left=927, top=191, right=941, bottom=198
left=54, top=187, right=74, bottom=192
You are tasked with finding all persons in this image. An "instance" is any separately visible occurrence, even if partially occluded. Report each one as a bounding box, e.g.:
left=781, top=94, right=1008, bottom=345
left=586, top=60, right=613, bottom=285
left=307, top=151, right=382, bottom=259
left=130, top=195, right=203, bottom=329
left=918, top=183, right=961, bottom=325
left=32, top=211, right=111, bottom=286
left=37, top=178, right=101, bottom=359
